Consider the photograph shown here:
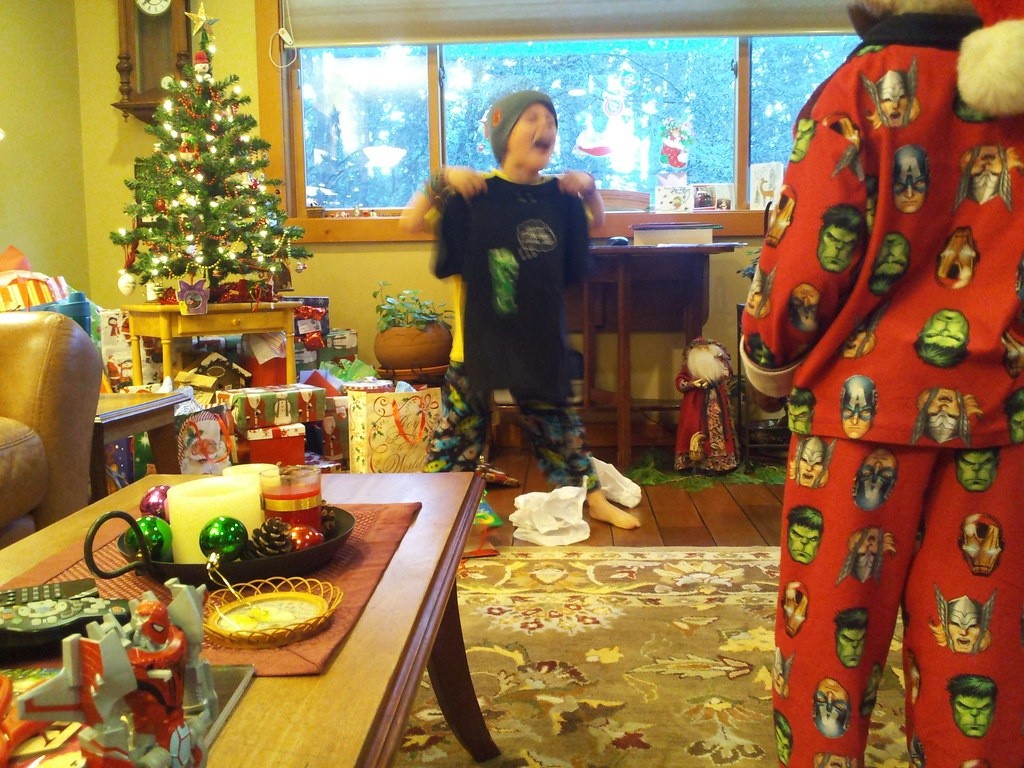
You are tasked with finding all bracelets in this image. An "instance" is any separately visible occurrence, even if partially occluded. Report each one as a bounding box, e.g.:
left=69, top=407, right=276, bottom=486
left=582, top=170, right=595, bottom=201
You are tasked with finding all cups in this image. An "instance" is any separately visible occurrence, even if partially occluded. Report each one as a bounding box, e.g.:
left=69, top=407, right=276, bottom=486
left=259, top=466, right=322, bottom=536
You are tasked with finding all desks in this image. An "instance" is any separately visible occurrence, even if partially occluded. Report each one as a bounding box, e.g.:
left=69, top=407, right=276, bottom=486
left=564, top=242, right=746, bottom=472
left=88, top=391, right=192, bottom=502
left=119, top=301, right=303, bottom=385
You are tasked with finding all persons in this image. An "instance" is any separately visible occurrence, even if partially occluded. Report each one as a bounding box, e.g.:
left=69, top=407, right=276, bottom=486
left=398, top=89, right=640, bottom=531
left=738, top=0, right=1024, bottom=767
left=675, top=336, right=740, bottom=476
left=17, top=576, right=221, bottom=768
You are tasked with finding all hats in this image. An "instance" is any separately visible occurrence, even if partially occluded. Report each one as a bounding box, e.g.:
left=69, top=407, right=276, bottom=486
left=487, top=90, right=558, bottom=165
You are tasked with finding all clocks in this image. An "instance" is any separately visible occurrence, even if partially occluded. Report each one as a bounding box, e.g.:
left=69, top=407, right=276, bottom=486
left=110, top=0, right=192, bottom=125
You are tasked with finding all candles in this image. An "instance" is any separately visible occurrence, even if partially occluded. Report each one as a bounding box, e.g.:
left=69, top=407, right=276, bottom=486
left=223, top=462, right=282, bottom=488
left=259, top=465, right=321, bottom=532
left=166, top=476, right=265, bottom=564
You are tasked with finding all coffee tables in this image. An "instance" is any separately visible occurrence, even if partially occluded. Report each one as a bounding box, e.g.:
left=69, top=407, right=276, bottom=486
left=0, top=472, right=503, bottom=768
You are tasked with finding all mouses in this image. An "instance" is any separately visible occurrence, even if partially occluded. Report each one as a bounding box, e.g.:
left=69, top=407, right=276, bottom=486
left=608, top=237, right=628, bottom=245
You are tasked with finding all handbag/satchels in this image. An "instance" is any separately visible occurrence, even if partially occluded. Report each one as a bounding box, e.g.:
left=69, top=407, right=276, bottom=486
left=133, top=405, right=231, bottom=483
left=462, top=496, right=504, bottom=558
left=347, top=385, right=442, bottom=474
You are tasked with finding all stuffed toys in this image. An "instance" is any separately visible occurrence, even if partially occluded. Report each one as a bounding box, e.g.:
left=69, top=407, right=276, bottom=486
left=193, top=51, right=213, bottom=82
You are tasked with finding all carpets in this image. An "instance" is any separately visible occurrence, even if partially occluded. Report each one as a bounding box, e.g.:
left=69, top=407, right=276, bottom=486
left=394, top=546, right=914, bottom=768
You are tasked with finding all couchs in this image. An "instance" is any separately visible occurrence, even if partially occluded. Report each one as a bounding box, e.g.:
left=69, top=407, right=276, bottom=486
left=0, top=310, right=103, bottom=550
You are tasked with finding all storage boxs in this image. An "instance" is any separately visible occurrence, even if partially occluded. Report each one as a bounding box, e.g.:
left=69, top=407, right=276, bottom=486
left=215, top=382, right=326, bottom=430
left=283, top=295, right=330, bottom=337
left=295, top=328, right=358, bottom=367
left=190, top=353, right=252, bottom=390
left=236, top=422, right=305, bottom=465
left=634, top=228, right=714, bottom=244
left=310, top=396, right=351, bottom=470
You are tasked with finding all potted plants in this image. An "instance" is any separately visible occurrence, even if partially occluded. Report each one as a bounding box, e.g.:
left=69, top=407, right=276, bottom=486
left=373, top=280, right=455, bottom=368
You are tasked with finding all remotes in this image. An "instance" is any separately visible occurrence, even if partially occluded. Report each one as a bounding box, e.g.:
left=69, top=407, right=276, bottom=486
left=0, top=599, right=132, bottom=665
left=0, top=578, right=99, bottom=607
